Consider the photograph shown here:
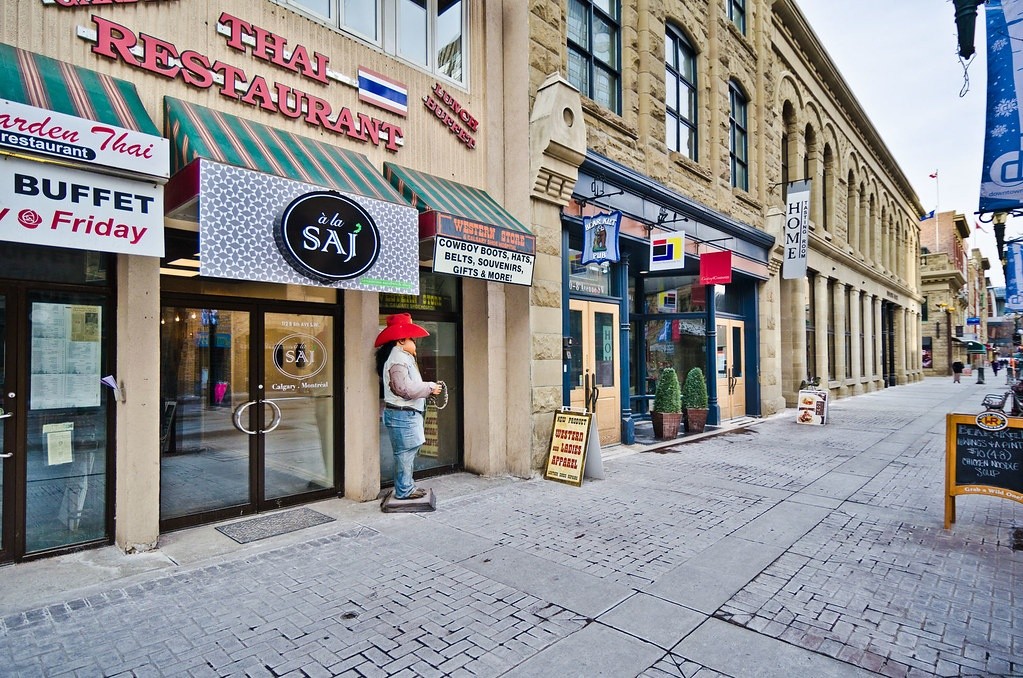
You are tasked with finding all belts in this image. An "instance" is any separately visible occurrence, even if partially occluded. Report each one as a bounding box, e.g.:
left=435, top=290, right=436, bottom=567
left=386, top=404, right=422, bottom=414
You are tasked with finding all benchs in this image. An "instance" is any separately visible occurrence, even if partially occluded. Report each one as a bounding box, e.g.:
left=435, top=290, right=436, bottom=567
left=981, top=392, right=1011, bottom=413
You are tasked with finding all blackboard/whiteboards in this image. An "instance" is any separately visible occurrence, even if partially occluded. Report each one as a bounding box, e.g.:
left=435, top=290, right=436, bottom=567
left=161, top=401, right=177, bottom=443
left=947, top=413, right=1023, bottom=503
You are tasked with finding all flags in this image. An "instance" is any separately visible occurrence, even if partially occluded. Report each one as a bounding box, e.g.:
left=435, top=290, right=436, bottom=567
left=976, top=223, right=981, bottom=229
left=920, top=210, right=935, bottom=220
left=928, top=169, right=937, bottom=178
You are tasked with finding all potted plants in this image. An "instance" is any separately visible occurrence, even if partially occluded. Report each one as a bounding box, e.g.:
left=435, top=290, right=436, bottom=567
left=682, top=367, right=710, bottom=433
left=649, top=367, right=683, bottom=441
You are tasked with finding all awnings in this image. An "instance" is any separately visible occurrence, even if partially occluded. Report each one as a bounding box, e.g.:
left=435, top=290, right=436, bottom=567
left=382, top=160, right=536, bottom=287
left=952, top=336, right=973, bottom=343
left=966, top=341, right=986, bottom=354
left=162, top=94, right=420, bottom=297
left=984, top=344, right=992, bottom=349
left=0, top=42, right=171, bottom=259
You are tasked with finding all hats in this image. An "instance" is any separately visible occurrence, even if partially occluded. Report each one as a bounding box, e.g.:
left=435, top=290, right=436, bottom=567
left=374, top=313, right=429, bottom=347
left=953, top=358, right=961, bottom=362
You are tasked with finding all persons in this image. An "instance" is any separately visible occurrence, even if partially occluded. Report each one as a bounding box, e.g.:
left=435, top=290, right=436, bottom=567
left=952, top=358, right=965, bottom=383
left=373, top=313, right=443, bottom=500
left=922, top=349, right=932, bottom=369
left=991, top=359, right=1009, bottom=376
